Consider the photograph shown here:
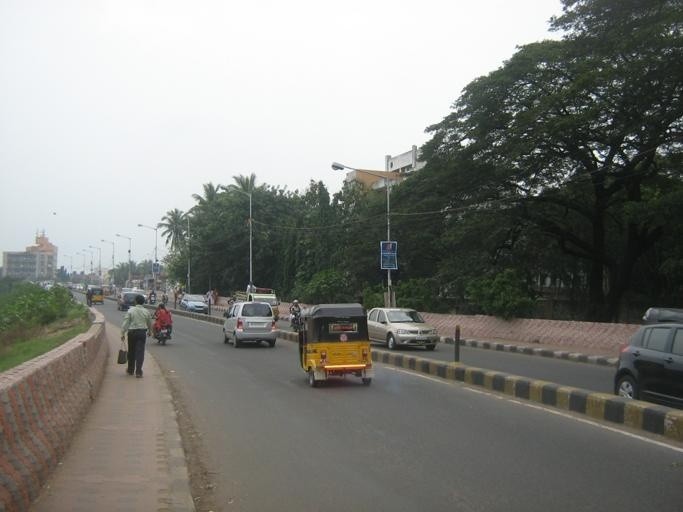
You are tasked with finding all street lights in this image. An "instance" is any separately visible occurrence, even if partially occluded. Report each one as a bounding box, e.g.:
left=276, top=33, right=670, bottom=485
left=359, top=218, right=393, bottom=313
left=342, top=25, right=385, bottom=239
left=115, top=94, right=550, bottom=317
left=220, top=184, right=256, bottom=293
left=75, top=223, right=157, bottom=291
left=331, top=162, right=396, bottom=307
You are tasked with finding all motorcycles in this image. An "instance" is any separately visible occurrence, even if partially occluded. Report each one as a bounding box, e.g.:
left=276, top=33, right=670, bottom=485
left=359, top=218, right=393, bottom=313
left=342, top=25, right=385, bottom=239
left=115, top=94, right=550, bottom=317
left=154, top=319, right=171, bottom=344
left=90, top=287, right=103, bottom=304
left=162, top=294, right=168, bottom=305
left=291, top=308, right=300, bottom=332
left=297, top=302, right=371, bottom=387
left=149, top=294, right=155, bottom=305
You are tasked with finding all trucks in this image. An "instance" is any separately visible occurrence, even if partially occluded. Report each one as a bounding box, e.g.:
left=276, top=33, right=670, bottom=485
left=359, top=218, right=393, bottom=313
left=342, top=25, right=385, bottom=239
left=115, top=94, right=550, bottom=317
left=230, top=291, right=279, bottom=321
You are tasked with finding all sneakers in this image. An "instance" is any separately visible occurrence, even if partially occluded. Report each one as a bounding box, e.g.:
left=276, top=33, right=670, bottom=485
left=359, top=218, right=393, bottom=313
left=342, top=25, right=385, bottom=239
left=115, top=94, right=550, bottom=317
left=127, top=368, right=142, bottom=378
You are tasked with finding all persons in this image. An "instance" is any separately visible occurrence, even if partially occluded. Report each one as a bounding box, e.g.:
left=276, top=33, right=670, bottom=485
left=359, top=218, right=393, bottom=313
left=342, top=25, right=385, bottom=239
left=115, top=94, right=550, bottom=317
left=287, top=298, right=301, bottom=321
left=120, top=293, right=155, bottom=378
left=206, top=287, right=216, bottom=304
left=85, top=288, right=93, bottom=305
left=148, top=289, right=169, bottom=303
left=151, top=303, right=175, bottom=333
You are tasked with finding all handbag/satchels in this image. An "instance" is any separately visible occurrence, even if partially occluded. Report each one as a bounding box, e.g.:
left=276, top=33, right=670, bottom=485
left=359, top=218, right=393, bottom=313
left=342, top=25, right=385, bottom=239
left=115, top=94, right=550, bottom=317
left=118, top=350, right=128, bottom=364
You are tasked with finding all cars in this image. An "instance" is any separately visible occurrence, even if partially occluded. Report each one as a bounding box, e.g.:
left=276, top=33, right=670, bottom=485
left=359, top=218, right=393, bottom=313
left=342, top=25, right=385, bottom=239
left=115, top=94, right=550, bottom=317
left=366, top=307, right=440, bottom=350
left=222, top=301, right=276, bottom=347
left=34, top=279, right=117, bottom=296
left=614, top=306, right=682, bottom=410
left=179, top=294, right=208, bottom=314
left=117, top=292, right=143, bottom=310
